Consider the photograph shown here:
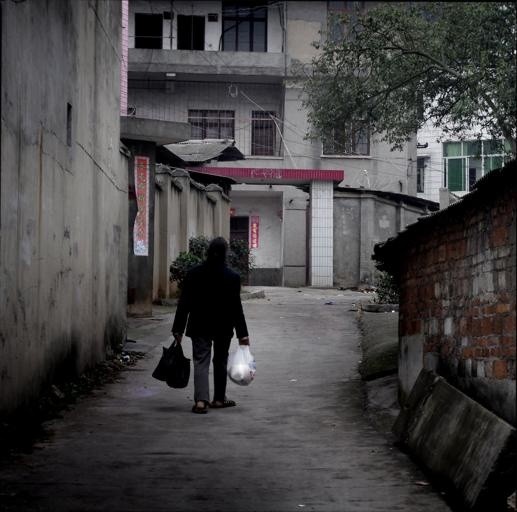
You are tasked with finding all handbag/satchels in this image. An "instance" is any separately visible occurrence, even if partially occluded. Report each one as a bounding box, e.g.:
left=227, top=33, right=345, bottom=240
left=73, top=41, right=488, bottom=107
left=152, top=346, right=190, bottom=388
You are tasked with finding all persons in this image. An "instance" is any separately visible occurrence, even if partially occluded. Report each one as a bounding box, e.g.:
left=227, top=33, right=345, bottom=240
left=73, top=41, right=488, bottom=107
left=170, top=235, right=251, bottom=415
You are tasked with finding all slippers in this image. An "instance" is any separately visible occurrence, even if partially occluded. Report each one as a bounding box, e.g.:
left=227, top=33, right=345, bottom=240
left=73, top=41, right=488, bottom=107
left=212, top=399, right=236, bottom=409
left=191, top=404, right=208, bottom=413
